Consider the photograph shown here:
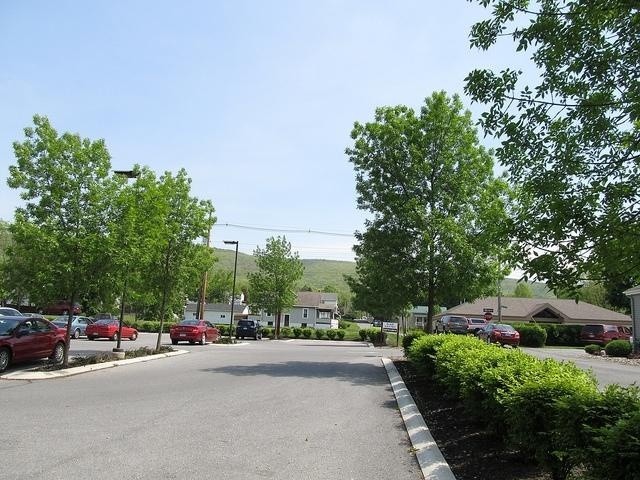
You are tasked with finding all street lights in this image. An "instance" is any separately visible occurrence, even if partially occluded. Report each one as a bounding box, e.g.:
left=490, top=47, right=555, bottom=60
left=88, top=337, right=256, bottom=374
left=223, top=241, right=238, bottom=338
left=114, top=170, right=140, bottom=352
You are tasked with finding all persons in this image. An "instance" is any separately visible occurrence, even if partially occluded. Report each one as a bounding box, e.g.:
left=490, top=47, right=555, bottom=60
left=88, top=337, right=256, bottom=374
left=255, top=320, right=264, bottom=341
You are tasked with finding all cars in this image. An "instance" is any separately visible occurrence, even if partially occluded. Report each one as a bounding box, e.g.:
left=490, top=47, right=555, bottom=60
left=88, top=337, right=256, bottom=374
left=353, top=316, right=381, bottom=327
left=236, top=319, right=262, bottom=340
left=0, top=299, right=138, bottom=373
left=170, top=319, right=221, bottom=345
left=432, top=314, right=520, bottom=348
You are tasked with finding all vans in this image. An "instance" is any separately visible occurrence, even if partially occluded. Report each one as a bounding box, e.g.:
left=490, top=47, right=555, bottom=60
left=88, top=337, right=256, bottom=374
left=579, top=323, right=632, bottom=347
